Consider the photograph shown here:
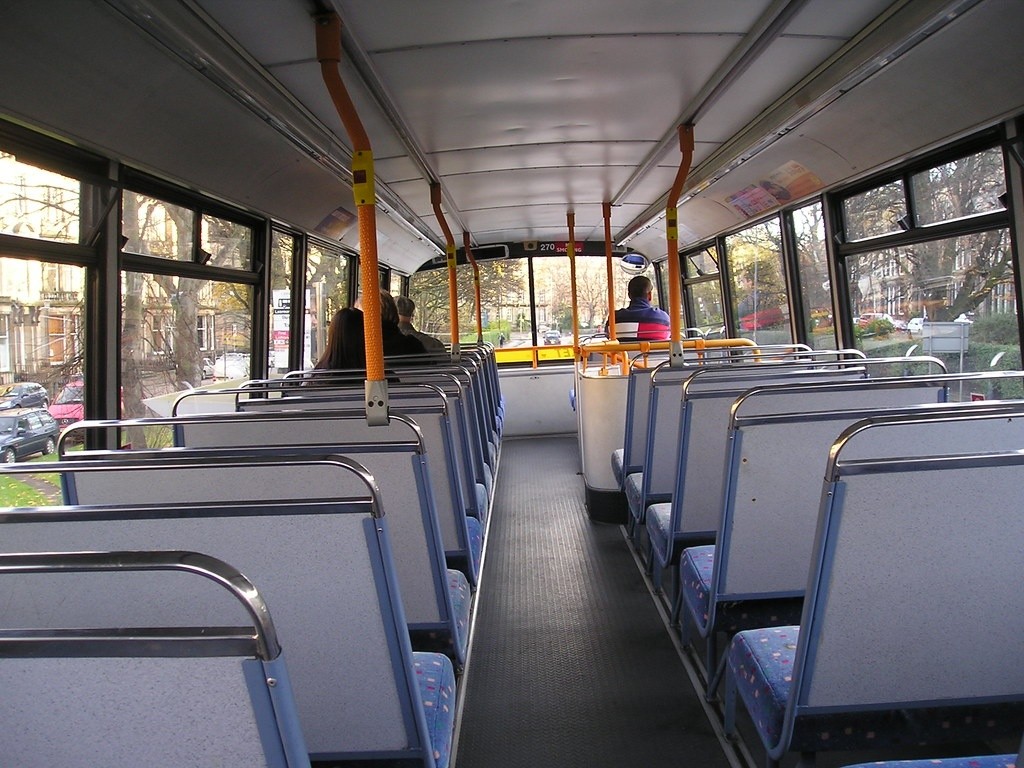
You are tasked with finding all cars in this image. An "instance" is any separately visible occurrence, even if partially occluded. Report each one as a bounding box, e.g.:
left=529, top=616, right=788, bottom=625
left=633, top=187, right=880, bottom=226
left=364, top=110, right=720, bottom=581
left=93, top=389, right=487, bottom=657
left=0, top=408, right=60, bottom=463
left=0, top=381, right=49, bottom=409
left=538, top=324, right=562, bottom=345
left=202, top=351, right=277, bottom=380
left=853, top=312, right=928, bottom=336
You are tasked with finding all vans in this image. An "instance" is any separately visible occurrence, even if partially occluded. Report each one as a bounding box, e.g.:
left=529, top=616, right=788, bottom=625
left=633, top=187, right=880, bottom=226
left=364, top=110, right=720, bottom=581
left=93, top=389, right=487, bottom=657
left=49, top=381, right=127, bottom=444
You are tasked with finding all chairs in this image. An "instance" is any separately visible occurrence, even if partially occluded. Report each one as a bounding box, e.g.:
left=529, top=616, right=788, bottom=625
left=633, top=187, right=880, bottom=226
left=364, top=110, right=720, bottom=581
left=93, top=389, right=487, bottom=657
left=57, top=411, right=475, bottom=675
left=0, top=453, right=457, bottom=768
left=569, top=323, right=949, bottom=627
left=0, top=550, right=313, bottom=768
left=838, top=732, right=1024, bottom=768
left=170, top=338, right=506, bottom=589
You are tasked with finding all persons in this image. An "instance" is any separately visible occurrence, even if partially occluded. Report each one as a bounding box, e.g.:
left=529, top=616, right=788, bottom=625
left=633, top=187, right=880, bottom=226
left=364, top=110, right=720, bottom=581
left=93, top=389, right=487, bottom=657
left=390, top=294, right=446, bottom=354
left=604, top=276, right=669, bottom=343
left=497, top=333, right=505, bottom=348
left=738, top=261, right=785, bottom=330
left=349, top=285, right=436, bottom=367
left=302, top=307, right=401, bottom=388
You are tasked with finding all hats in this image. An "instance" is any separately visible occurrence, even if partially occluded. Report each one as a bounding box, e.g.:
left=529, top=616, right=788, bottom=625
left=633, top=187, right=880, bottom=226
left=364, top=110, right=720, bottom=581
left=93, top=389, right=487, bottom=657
left=628, top=276, right=653, bottom=295
left=392, top=296, right=415, bottom=316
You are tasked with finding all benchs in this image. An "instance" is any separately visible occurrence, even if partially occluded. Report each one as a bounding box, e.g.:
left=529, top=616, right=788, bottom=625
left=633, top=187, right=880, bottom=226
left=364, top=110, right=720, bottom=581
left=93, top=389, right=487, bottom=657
left=677, top=371, right=1024, bottom=663
left=706, top=398, right=1024, bottom=768
